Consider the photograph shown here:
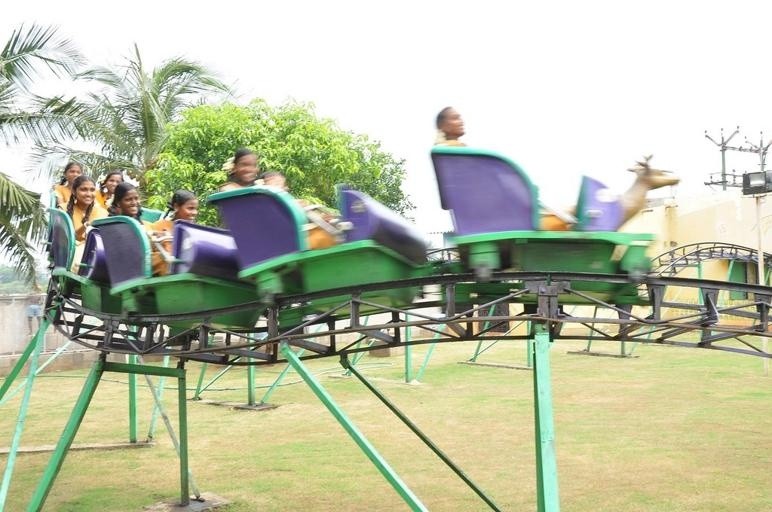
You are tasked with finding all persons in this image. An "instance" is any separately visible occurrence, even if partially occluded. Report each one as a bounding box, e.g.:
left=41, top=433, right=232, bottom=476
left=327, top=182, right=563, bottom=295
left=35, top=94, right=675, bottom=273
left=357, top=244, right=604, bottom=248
left=434, top=106, right=468, bottom=147
left=52, top=148, right=346, bottom=276
left=23, top=283, right=44, bottom=337
